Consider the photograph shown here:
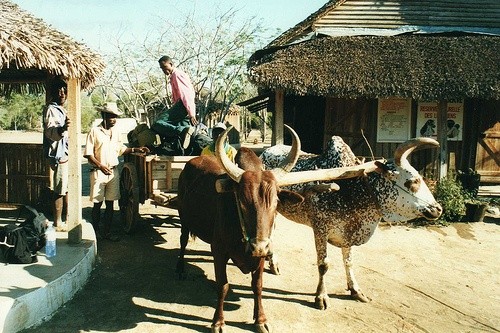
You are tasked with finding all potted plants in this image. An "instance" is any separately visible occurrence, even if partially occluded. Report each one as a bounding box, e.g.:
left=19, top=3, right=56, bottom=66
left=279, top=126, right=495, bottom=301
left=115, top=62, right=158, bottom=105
left=464, top=199, right=488, bottom=222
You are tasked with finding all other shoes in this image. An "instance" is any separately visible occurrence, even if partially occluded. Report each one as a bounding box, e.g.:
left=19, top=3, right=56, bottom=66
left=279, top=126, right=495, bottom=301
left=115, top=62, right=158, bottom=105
left=102, top=231, right=119, bottom=242
left=96, top=231, right=103, bottom=240
left=53, top=225, right=69, bottom=232
left=179, top=126, right=196, bottom=150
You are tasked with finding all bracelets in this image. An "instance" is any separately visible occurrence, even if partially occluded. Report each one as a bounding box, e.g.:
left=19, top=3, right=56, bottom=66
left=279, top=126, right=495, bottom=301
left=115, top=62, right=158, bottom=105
left=131, top=147, right=135, bottom=153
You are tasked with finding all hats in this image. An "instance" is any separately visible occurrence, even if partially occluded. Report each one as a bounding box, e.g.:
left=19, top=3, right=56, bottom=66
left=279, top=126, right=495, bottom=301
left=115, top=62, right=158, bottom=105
left=94, top=102, right=124, bottom=118
left=214, top=122, right=227, bottom=132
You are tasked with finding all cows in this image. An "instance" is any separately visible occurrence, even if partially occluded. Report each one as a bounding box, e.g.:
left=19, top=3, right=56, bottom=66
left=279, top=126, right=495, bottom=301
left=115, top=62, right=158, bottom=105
left=259, top=136, right=442, bottom=310
left=178, top=124, right=301, bottom=333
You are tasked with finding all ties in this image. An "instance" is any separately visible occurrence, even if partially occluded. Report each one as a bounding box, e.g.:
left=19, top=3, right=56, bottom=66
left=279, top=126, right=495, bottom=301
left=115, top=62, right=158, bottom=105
left=93, top=166, right=116, bottom=171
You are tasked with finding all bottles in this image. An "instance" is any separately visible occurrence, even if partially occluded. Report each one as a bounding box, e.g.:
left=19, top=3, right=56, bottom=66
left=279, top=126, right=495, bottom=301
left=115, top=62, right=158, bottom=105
left=46, top=222, right=56, bottom=257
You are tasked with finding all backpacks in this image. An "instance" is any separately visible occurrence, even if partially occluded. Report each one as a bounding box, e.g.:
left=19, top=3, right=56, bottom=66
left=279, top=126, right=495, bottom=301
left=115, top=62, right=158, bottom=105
left=0, top=203, right=48, bottom=264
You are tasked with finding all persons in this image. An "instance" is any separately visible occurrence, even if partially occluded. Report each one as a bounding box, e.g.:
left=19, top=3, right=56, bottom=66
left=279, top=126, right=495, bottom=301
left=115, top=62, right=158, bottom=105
left=151, top=56, right=239, bottom=166
left=43, top=77, right=71, bottom=233
left=83, top=102, right=150, bottom=243
